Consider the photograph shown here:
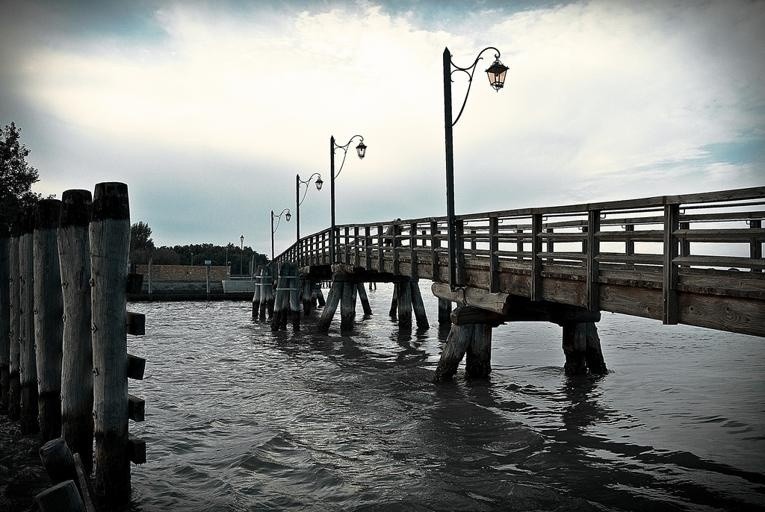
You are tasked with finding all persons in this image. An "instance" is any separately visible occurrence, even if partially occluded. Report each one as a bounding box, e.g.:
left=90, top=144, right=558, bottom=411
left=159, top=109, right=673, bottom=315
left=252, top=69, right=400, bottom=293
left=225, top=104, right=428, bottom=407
left=385, top=219, right=401, bottom=252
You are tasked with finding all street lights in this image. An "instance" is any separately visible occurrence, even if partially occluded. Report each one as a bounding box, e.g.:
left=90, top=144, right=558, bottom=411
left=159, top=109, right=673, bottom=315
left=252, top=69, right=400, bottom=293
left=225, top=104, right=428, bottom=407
left=271, top=208, right=292, bottom=261
left=296, top=173, right=323, bottom=242
left=240, top=235, right=244, bottom=276
left=443, top=45, right=509, bottom=290
left=330, top=134, right=367, bottom=264
